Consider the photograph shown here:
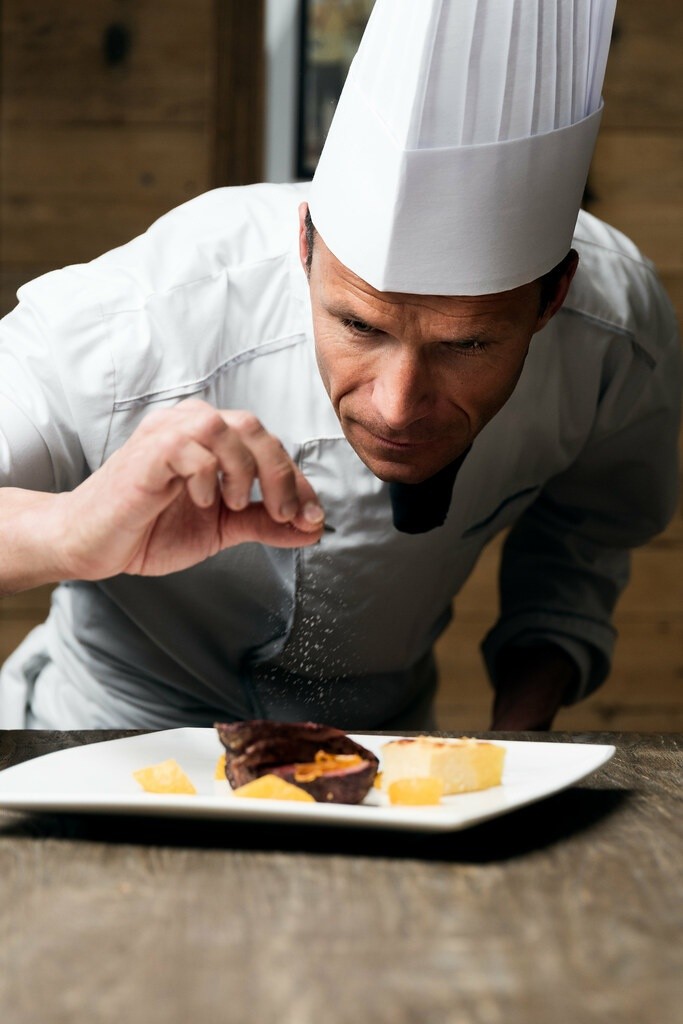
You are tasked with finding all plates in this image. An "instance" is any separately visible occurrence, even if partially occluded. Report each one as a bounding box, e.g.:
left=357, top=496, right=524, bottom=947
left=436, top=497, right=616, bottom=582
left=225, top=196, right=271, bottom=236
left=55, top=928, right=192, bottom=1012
left=0, top=727, right=617, bottom=831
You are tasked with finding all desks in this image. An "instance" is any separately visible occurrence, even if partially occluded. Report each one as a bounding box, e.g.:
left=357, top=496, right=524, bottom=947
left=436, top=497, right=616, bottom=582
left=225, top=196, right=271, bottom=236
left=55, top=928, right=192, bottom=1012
left=0, top=729, right=683, bottom=1024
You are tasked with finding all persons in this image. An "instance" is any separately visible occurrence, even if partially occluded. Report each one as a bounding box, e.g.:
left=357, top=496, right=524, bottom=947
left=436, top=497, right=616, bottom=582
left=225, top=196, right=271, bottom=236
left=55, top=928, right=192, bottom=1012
left=1, top=1, right=682, bottom=732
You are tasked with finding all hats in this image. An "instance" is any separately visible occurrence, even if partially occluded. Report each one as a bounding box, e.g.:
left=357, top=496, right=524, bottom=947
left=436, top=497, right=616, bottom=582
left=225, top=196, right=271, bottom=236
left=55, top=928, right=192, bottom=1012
left=303, top=1, right=617, bottom=296
